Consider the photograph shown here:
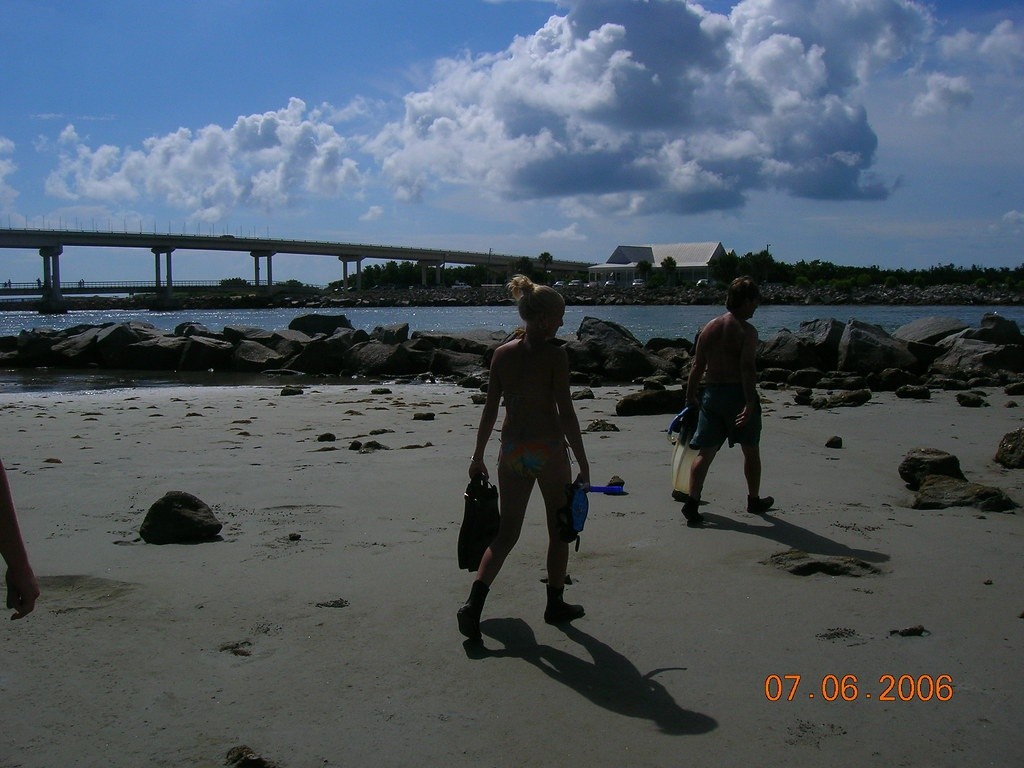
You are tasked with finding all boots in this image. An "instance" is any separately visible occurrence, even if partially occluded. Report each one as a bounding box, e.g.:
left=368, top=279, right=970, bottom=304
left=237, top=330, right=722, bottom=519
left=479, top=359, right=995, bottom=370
left=457, top=580, right=490, bottom=641
left=544, top=584, right=583, bottom=624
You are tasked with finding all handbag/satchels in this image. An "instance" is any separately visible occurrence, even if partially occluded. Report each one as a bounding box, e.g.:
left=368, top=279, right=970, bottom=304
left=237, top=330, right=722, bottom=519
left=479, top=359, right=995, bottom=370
left=457, top=473, right=500, bottom=572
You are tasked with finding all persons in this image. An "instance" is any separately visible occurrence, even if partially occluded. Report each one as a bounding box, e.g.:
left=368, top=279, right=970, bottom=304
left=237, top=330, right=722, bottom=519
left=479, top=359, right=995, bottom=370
left=0, top=458, right=41, bottom=621
left=680, top=276, right=774, bottom=521
left=455, top=274, right=592, bottom=639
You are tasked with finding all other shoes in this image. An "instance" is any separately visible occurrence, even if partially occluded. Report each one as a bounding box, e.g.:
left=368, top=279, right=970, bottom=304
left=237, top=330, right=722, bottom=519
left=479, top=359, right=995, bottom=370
left=681, top=496, right=704, bottom=522
left=747, top=495, right=774, bottom=513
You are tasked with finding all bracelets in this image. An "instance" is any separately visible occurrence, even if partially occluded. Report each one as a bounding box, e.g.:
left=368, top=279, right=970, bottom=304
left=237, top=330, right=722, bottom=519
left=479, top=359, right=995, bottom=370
left=470, top=456, right=483, bottom=464
